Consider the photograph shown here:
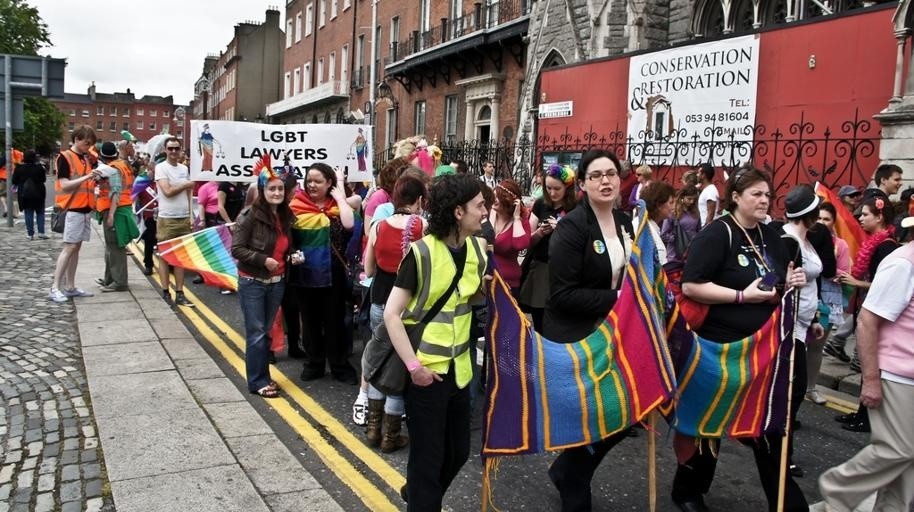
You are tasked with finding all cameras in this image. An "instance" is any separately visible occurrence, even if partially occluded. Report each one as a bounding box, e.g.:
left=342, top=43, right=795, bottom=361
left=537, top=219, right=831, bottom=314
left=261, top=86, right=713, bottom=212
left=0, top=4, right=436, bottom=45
left=291, top=251, right=306, bottom=263
left=758, top=272, right=779, bottom=292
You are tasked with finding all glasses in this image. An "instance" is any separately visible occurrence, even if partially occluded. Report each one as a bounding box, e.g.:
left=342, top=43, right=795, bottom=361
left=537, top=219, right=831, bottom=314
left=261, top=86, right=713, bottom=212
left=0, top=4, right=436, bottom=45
left=168, top=146, right=181, bottom=151
left=584, top=172, right=619, bottom=181
left=847, top=193, right=860, bottom=198
left=636, top=174, right=642, bottom=176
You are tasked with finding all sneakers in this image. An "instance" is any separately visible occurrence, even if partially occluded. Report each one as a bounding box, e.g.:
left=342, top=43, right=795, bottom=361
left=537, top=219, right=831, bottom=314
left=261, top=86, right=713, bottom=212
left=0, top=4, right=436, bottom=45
left=100, top=284, right=128, bottom=292
left=850, top=358, right=862, bottom=372
left=38, top=233, right=50, bottom=239
left=301, top=363, right=324, bottom=381
left=95, top=278, right=108, bottom=286
left=144, top=267, right=151, bottom=275
left=334, top=366, right=359, bottom=385
left=26, top=235, right=33, bottom=240
left=806, top=388, right=827, bottom=404
left=789, top=462, right=802, bottom=477
left=175, top=294, right=195, bottom=307
left=353, top=398, right=368, bottom=425
left=676, top=500, right=710, bottom=512
left=50, top=288, right=68, bottom=302
left=163, top=294, right=172, bottom=307
left=824, top=339, right=850, bottom=363
left=64, top=288, right=94, bottom=297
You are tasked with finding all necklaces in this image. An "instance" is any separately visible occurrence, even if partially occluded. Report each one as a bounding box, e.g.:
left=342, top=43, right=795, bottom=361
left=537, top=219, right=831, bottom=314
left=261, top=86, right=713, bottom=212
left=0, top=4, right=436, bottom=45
left=493, top=215, right=510, bottom=238
left=730, top=212, right=772, bottom=274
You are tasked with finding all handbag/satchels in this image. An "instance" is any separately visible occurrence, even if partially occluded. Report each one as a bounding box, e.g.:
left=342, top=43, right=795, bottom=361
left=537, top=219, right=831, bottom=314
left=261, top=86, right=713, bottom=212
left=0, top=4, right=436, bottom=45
left=330, top=243, right=357, bottom=287
left=361, top=245, right=468, bottom=396
left=669, top=218, right=732, bottom=331
left=51, top=163, right=87, bottom=233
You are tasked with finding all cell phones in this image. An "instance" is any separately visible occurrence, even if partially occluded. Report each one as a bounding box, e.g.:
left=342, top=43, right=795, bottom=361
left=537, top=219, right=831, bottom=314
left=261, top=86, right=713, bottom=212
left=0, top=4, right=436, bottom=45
left=543, top=219, right=552, bottom=224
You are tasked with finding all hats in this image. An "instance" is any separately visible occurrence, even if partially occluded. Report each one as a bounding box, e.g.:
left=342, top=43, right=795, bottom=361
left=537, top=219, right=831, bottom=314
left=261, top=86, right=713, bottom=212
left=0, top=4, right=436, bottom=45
left=21, top=149, right=39, bottom=163
left=901, top=216, right=914, bottom=228
left=99, top=141, right=119, bottom=158
left=838, top=185, right=860, bottom=195
left=784, top=185, right=825, bottom=219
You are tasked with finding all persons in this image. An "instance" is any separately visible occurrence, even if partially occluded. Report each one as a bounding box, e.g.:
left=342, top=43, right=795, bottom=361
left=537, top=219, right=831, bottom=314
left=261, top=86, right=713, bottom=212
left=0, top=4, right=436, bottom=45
left=154, top=137, right=198, bottom=308
left=135, top=151, right=175, bottom=276
left=346, top=173, right=369, bottom=202
left=619, top=159, right=639, bottom=212
left=353, top=157, right=407, bottom=426
left=140, top=152, right=151, bottom=176
left=817, top=240, right=914, bottom=512
left=833, top=196, right=900, bottom=433
left=364, top=177, right=429, bottom=455
left=805, top=202, right=850, bottom=404
left=119, top=142, right=141, bottom=176
left=631, top=180, right=676, bottom=265
left=765, top=183, right=837, bottom=479
left=199, top=124, right=223, bottom=172
left=474, top=180, right=496, bottom=245
left=670, top=167, right=810, bottom=512
left=288, top=163, right=362, bottom=386
left=492, top=178, right=539, bottom=302
left=230, top=171, right=305, bottom=398
left=449, top=158, right=469, bottom=176
left=837, top=183, right=862, bottom=214
left=660, top=183, right=700, bottom=263
left=629, top=165, right=652, bottom=218
left=823, top=165, right=904, bottom=372
left=12, top=150, right=50, bottom=240
left=520, top=163, right=576, bottom=334
left=47, top=124, right=98, bottom=303
left=545, top=148, right=645, bottom=512
left=95, top=141, right=140, bottom=293
left=383, top=173, right=495, bottom=512
left=347, top=129, right=369, bottom=171
left=216, top=180, right=246, bottom=295
left=238, top=183, right=307, bottom=364
left=696, top=162, right=720, bottom=228
left=132, top=155, right=141, bottom=177
left=193, top=181, right=220, bottom=285
left=679, top=169, right=698, bottom=187
left=478, top=161, right=497, bottom=191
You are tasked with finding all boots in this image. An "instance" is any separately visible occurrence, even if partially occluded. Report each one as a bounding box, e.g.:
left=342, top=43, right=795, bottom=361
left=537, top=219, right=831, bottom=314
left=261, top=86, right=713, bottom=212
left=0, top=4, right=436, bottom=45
left=381, top=413, right=409, bottom=453
left=367, top=398, right=385, bottom=442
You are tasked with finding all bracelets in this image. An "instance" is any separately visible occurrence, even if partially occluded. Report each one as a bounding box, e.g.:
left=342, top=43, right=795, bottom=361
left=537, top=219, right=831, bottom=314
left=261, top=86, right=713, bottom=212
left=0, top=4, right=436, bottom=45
left=739, top=290, right=743, bottom=303
left=736, top=290, right=739, bottom=303
left=406, top=361, right=420, bottom=371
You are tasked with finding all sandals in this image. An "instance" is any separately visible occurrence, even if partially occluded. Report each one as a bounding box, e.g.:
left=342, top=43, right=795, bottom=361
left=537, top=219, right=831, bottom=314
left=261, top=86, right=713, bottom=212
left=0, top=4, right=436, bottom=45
left=834, top=413, right=858, bottom=423
left=842, top=421, right=871, bottom=432
left=269, top=380, right=281, bottom=391
left=257, top=385, right=279, bottom=397
